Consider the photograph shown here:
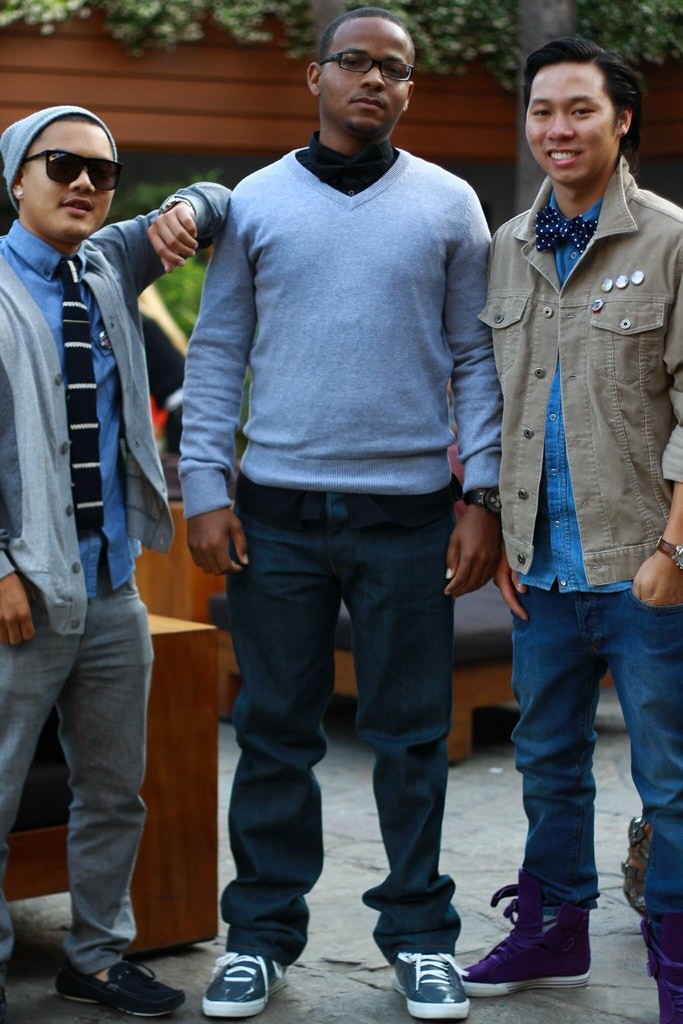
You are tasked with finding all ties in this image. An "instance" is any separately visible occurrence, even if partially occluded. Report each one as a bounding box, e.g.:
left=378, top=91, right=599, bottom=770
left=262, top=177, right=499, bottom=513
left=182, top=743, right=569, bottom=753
left=54, top=259, right=104, bottom=534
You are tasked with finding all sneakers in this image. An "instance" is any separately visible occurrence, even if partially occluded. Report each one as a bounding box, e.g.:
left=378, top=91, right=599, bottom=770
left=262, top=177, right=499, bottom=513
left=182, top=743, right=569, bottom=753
left=393, top=953, right=470, bottom=1018
left=202, top=953, right=289, bottom=1017
left=462, top=869, right=591, bottom=997
left=55, top=961, right=185, bottom=1016
left=640, top=911, right=683, bottom=1024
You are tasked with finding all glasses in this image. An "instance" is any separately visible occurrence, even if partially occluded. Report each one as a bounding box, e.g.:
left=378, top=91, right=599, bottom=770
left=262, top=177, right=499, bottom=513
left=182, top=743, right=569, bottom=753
left=320, top=51, right=414, bottom=81
left=23, top=150, right=123, bottom=190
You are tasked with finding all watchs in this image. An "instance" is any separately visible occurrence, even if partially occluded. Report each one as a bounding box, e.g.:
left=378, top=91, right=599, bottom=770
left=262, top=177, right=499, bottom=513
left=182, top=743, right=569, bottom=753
left=462, top=488, right=502, bottom=520
left=656, top=536, right=683, bottom=571
left=158, top=196, right=193, bottom=216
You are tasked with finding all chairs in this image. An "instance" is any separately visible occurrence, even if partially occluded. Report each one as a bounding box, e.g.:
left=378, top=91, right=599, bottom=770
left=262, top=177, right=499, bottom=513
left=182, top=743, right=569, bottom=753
left=3, top=612, right=220, bottom=957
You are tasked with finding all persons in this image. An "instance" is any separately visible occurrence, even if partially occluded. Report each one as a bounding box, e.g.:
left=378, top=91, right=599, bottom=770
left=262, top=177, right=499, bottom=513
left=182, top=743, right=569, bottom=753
left=173, top=1, right=502, bottom=1020
left=454, top=38, right=683, bottom=1024
left=0, top=105, right=231, bottom=1024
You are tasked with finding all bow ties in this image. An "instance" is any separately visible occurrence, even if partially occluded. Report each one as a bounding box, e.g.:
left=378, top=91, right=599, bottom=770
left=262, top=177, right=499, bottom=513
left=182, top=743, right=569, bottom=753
left=535, top=206, right=598, bottom=254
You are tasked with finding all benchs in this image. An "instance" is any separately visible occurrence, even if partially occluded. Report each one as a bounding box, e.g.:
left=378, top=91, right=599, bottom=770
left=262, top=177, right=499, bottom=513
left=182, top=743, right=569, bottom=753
left=209, top=573, right=608, bottom=760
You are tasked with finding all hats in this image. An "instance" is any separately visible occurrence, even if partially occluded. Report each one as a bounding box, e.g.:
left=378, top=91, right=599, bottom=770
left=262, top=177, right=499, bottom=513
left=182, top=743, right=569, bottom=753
left=0, top=105, right=118, bottom=213
